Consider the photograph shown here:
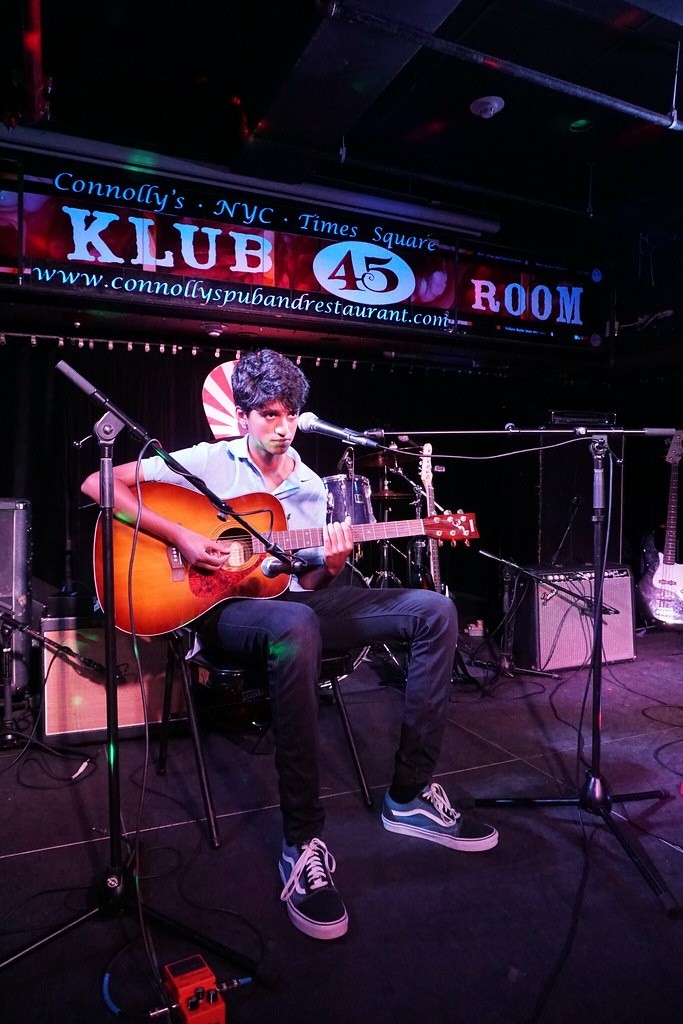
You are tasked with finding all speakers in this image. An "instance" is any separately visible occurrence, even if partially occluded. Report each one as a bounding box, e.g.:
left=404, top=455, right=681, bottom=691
left=511, top=562, right=637, bottom=672
left=40, top=595, right=201, bottom=748
left=0, top=498, right=34, bottom=696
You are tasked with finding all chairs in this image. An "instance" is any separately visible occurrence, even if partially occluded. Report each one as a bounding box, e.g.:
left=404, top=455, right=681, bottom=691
left=154, top=625, right=373, bottom=851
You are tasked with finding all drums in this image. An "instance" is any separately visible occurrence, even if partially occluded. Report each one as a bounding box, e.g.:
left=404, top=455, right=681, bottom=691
left=322, top=473, right=378, bottom=525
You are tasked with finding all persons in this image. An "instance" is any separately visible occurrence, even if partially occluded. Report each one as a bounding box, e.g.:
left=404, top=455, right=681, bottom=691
left=81, top=350, right=497, bottom=938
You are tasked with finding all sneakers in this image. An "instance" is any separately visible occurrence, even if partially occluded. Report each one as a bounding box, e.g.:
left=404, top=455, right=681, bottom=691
left=278, top=836, right=348, bottom=940
left=380, top=783, right=499, bottom=852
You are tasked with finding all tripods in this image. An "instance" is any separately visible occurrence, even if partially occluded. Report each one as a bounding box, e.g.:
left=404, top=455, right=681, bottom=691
left=0, top=611, right=109, bottom=760
left=0, top=360, right=296, bottom=1009
left=356, top=425, right=683, bottom=922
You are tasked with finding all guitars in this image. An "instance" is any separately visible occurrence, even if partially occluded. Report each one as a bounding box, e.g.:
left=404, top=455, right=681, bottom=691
left=418, top=441, right=442, bottom=594
left=91, top=480, right=481, bottom=643
left=635, top=430, right=683, bottom=629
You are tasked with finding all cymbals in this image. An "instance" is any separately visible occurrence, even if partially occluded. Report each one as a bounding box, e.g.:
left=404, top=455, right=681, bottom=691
left=354, top=446, right=421, bottom=467
left=370, top=489, right=411, bottom=499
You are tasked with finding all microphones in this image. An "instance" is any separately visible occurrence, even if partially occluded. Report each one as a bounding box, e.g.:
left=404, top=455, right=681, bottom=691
left=398, top=435, right=422, bottom=453
left=298, top=412, right=378, bottom=449
left=337, top=446, right=351, bottom=470
left=602, top=608, right=619, bottom=615
left=260, top=557, right=304, bottom=578
left=112, top=669, right=128, bottom=683
